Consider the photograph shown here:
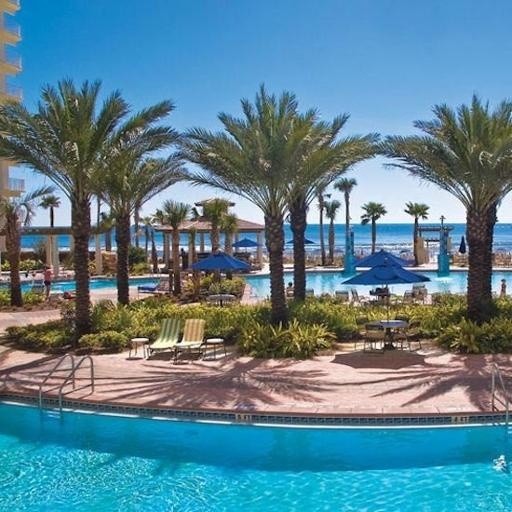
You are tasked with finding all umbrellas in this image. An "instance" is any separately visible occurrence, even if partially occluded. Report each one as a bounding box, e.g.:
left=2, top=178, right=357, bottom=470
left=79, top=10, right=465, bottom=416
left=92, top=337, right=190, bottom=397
left=341, top=260, right=431, bottom=323
left=457, top=235, right=467, bottom=255
left=350, top=247, right=412, bottom=270
left=230, top=237, right=263, bottom=252
left=286, top=235, right=314, bottom=245
left=189, top=251, right=252, bottom=295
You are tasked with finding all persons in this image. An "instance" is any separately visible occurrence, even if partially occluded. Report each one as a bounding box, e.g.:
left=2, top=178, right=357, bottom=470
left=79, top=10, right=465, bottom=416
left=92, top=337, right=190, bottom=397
left=499, top=279, right=507, bottom=298
left=43, top=265, right=52, bottom=298
left=418, top=285, right=429, bottom=300
left=374, top=286, right=388, bottom=293
left=286, top=281, right=294, bottom=291
left=62, top=290, right=78, bottom=299
left=179, top=246, right=187, bottom=267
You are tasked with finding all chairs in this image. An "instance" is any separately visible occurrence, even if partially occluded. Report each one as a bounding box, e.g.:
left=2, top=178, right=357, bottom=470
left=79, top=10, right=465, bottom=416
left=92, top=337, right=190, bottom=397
left=146, top=317, right=206, bottom=366
left=354, top=313, right=423, bottom=354
left=335, top=283, right=428, bottom=305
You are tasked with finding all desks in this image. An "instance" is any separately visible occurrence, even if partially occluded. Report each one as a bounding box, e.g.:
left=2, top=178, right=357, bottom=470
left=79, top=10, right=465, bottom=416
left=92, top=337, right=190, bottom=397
left=128, top=337, right=149, bottom=359
left=209, top=294, right=235, bottom=307
left=206, top=338, right=226, bottom=361
